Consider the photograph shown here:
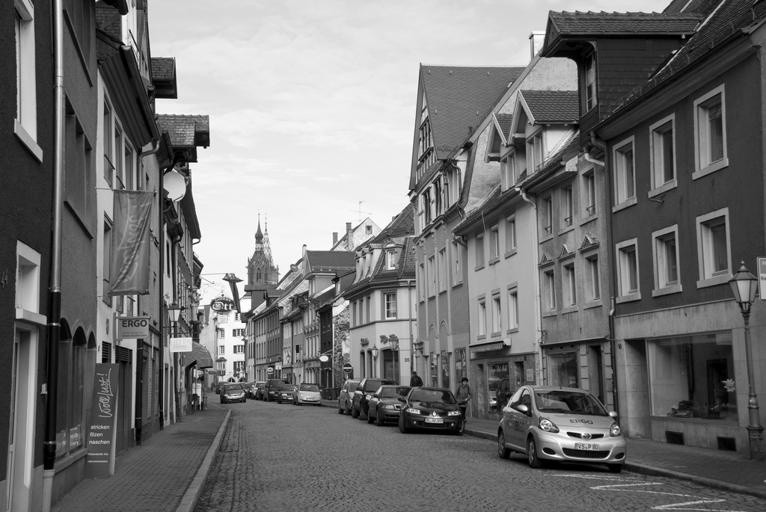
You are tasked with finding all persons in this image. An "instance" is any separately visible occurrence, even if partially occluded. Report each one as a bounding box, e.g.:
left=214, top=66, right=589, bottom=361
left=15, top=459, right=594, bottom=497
left=454, top=377, right=472, bottom=422
left=410, top=371, right=423, bottom=386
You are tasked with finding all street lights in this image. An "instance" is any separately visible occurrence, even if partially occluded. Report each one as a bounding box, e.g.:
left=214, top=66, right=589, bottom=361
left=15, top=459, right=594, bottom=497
left=728, top=260, right=765, bottom=461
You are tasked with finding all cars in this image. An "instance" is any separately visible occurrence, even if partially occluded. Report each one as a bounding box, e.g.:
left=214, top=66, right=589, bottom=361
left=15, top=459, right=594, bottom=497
left=366, top=384, right=412, bottom=426
left=351, top=377, right=397, bottom=419
left=495, top=384, right=627, bottom=473
left=338, top=379, right=361, bottom=415
left=220, top=377, right=322, bottom=407
left=397, top=386, right=465, bottom=435
